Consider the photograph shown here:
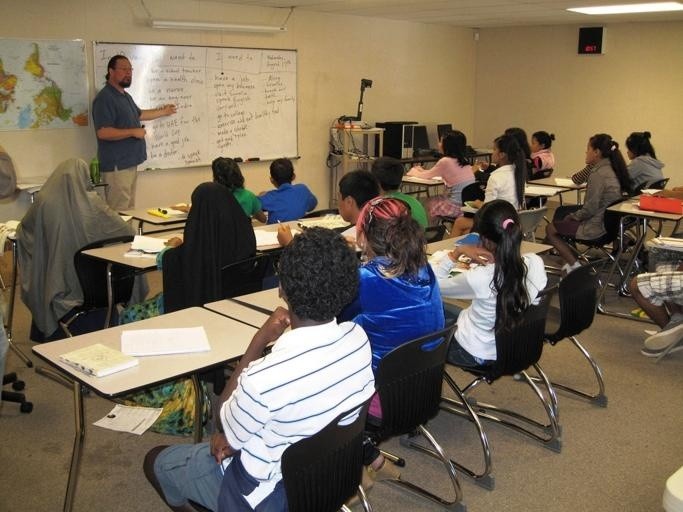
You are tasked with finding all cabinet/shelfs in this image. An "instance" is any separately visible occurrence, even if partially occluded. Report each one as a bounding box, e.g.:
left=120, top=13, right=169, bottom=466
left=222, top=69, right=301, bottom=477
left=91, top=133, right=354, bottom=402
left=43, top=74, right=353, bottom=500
left=329, top=127, right=383, bottom=211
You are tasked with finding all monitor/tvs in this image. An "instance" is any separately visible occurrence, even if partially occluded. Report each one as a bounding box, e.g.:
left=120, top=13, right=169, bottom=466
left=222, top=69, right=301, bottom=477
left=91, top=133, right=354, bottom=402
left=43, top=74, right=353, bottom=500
left=436, top=123, right=452, bottom=142
left=409, top=124, right=429, bottom=157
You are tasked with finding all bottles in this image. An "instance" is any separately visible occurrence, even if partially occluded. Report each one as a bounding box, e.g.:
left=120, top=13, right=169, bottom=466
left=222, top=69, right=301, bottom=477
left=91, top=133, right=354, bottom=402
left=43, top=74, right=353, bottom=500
left=90, top=159, right=101, bottom=184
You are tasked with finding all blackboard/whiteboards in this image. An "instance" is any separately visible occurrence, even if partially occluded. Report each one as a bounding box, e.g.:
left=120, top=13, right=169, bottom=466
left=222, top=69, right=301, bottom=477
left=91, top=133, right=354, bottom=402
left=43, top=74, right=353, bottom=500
left=93, top=38, right=301, bottom=173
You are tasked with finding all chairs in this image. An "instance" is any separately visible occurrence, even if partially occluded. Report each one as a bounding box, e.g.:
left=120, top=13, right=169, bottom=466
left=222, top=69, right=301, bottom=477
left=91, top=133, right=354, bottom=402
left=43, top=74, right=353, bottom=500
left=437, top=284, right=559, bottom=445
left=511, top=255, right=608, bottom=401
left=186, top=388, right=379, bottom=511
left=366, top=324, right=492, bottom=507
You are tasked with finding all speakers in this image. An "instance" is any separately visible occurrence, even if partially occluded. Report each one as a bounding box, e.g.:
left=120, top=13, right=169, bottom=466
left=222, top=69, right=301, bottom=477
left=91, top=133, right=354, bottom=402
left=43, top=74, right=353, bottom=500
left=375, top=119, right=414, bottom=159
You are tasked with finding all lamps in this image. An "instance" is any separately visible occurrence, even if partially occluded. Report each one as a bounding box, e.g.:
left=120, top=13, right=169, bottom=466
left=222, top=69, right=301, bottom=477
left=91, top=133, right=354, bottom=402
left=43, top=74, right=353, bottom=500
left=141, top=0, right=295, bottom=33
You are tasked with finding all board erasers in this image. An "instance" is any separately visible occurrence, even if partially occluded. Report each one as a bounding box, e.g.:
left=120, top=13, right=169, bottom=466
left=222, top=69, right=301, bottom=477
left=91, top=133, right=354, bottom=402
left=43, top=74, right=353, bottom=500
left=247, top=156, right=260, bottom=162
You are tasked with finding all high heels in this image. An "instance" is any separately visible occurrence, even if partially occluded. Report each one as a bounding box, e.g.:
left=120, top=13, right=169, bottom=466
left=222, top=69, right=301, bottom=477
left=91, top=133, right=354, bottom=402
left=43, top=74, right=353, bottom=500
left=367, top=454, right=401, bottom=482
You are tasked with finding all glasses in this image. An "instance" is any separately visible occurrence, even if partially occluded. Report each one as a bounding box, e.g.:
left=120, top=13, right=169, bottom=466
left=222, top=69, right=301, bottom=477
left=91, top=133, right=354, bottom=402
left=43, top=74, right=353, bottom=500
left=115, top=67, right=134, bottom=72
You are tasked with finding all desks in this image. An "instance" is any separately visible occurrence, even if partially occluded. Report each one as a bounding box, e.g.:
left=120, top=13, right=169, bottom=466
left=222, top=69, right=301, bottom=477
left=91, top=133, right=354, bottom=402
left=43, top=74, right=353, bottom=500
left=596, top=198, right=683, bottom=324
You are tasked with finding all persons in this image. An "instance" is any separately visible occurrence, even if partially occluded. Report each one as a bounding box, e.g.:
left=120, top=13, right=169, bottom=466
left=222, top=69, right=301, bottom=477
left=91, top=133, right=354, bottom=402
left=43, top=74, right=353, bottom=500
left=406, top=130, right=475, bottom=227
left=371, top=156, right=428, bottom=229
left=449, top=135, right=527, bottom=238
left=337, top=196, right=445, bottom=508
left=105, top=182, right=256, bottom=437
left=16, top=157, right=137, bottom=344
left=257, top=158, right=318, bottom=225
left=652, top=185, right=683, bottom=200
left=630, top=236, right=683, bottom=359
left=277, top=170, right=380, bottom=252
left=169, top=156, right=267, bottom=223
left=545, top=133, right=636, bottom=284
left=0, top=145, right=34, bottom=224
left=143, top=226, right=375, bottom=511
left=610, top=131, right=665, bottom=256
left=461, top=127, right=533, bottom=220
left=527, top=131, right=555, bottom=209
left=435, top=199, right=548, bottom=369
left=0, top=311, right=10, bottom=409
left=551, top=164, right=593, bottom=222
left=92, top=54, right=177, bottom=212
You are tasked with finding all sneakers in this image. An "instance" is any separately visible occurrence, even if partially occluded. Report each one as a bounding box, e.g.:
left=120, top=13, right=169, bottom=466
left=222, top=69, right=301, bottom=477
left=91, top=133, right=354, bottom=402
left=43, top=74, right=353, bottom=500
left=641, top=339, right=683, bottom=358
left=644, top=319, right=683, bottom=352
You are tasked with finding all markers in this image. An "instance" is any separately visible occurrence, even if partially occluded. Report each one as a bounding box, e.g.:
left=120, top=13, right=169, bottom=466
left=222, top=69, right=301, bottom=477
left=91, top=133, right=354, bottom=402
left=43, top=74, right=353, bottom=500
left=158, top=208, right=167, bottom=214
left=297, top=223, right=308, bottom=230
left=145, top=168, right=160, bottom=170
